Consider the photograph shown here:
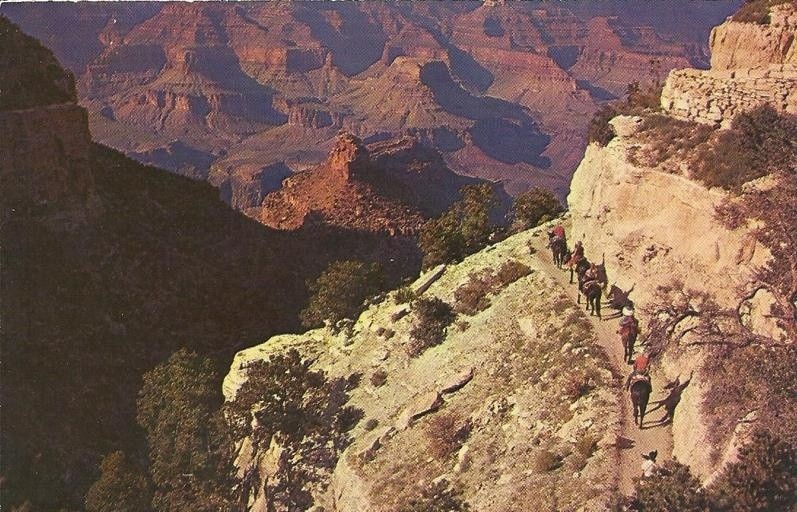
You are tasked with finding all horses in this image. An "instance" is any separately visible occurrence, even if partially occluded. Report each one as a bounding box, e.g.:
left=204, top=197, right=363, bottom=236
left=629, top=365, right=652, bottom=431
left=546, top=230, right=567, bottom=270
left=563, top=248, right=591, bottom=285
left=619, top=324, right=637, bottom=363
left=576, top=266, right=603, bottom=320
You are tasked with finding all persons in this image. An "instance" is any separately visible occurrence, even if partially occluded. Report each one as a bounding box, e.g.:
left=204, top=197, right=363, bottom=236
left=565, top=240, right=584, bottom=268
left=545, top=220, right=566, bottom=250
left=640, top=449, right=658, bottom=480
left=615, top=305, right=641, bottom=335
left=622, top=349, right=654, bottom=393
left=577, top=262, right=598, bottom=290
